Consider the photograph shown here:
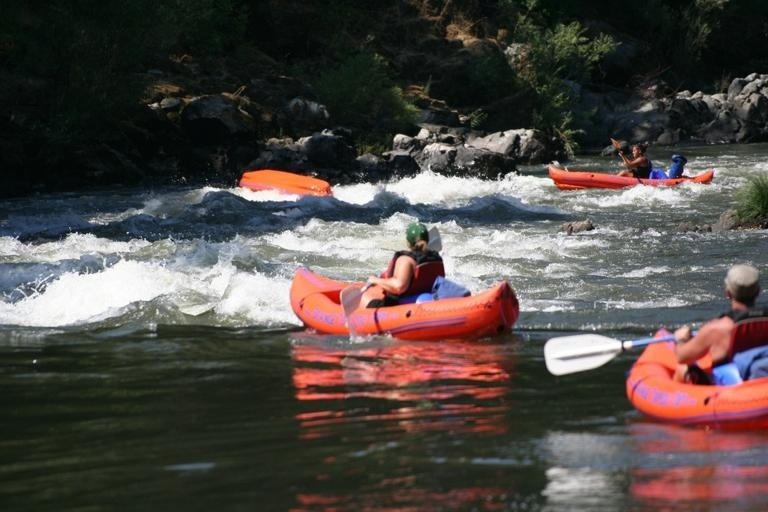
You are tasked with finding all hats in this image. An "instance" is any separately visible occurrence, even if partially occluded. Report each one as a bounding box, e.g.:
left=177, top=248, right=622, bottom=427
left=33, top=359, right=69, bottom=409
left=724, top=264, right=761, bottom=298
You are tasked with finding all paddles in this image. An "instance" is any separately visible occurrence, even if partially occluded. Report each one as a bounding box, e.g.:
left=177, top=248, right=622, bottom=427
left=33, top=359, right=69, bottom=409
left=340, top=226, right=443, bottom=317
left=544, top=330, right=700, bottom=376
left=609, top=139, right=631, bottom=174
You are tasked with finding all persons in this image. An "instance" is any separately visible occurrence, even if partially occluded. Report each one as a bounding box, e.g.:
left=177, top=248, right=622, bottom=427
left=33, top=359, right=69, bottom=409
left=668, top=263, right=767, bottom=386
left=363, top=222, right=445, bottom=310
left=618, top=144, right=653, bottom=178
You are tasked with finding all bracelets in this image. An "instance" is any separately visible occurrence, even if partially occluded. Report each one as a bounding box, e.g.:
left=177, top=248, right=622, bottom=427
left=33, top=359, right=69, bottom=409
left=675, top=339, right=687, bottom=344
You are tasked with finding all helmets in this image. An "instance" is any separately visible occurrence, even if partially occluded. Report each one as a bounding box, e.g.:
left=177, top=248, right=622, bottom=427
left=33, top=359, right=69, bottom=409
left=405, top=222, right=429, bottom=245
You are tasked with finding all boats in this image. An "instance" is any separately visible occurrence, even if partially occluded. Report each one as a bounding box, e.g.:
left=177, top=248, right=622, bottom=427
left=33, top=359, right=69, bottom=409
left=288, top=265, right=519, bottom=341
left=239, top=170, right=333, bottom=198
left=625, top=325, right=768, bottom=430
left=548, top=162, right=715, bottom=190
